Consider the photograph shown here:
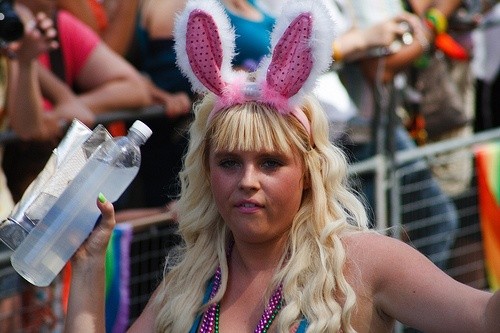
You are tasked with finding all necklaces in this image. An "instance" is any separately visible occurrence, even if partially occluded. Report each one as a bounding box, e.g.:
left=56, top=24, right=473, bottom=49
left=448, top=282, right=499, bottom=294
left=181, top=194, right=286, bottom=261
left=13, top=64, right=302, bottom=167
left=197, top=265, right=285, bottom=333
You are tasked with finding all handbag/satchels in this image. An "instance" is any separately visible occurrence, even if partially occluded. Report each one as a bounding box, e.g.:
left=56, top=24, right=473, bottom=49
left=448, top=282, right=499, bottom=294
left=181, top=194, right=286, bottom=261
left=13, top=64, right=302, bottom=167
left=413, top=16, right=476, bottom=139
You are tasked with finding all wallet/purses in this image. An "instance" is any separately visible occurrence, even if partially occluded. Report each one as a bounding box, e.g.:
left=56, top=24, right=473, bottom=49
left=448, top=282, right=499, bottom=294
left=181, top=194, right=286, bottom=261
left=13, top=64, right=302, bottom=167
left=0, top=118, right=125, bottom=268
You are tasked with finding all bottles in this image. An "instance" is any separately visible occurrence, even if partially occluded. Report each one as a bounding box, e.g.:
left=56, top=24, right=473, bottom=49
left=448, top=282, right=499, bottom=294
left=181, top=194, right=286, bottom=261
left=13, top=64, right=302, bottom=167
left=10, top=120, right=153, bottom=286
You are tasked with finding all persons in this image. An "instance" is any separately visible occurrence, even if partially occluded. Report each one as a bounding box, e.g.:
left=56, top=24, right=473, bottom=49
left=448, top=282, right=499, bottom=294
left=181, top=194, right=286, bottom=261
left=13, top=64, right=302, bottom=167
left=65, top=0, right=500, bottom=333
left=0, top=0, right=500, bottom=272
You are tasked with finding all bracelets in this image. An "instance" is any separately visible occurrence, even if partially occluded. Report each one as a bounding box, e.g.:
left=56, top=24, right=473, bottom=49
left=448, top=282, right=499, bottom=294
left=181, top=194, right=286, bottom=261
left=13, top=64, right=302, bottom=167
left=331, top=39, right=343, bottom=62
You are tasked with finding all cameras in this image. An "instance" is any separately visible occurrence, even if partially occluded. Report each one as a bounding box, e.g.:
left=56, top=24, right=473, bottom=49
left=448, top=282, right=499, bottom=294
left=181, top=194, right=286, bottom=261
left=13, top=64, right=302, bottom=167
left=0, top=0, right=24, bottom=43
